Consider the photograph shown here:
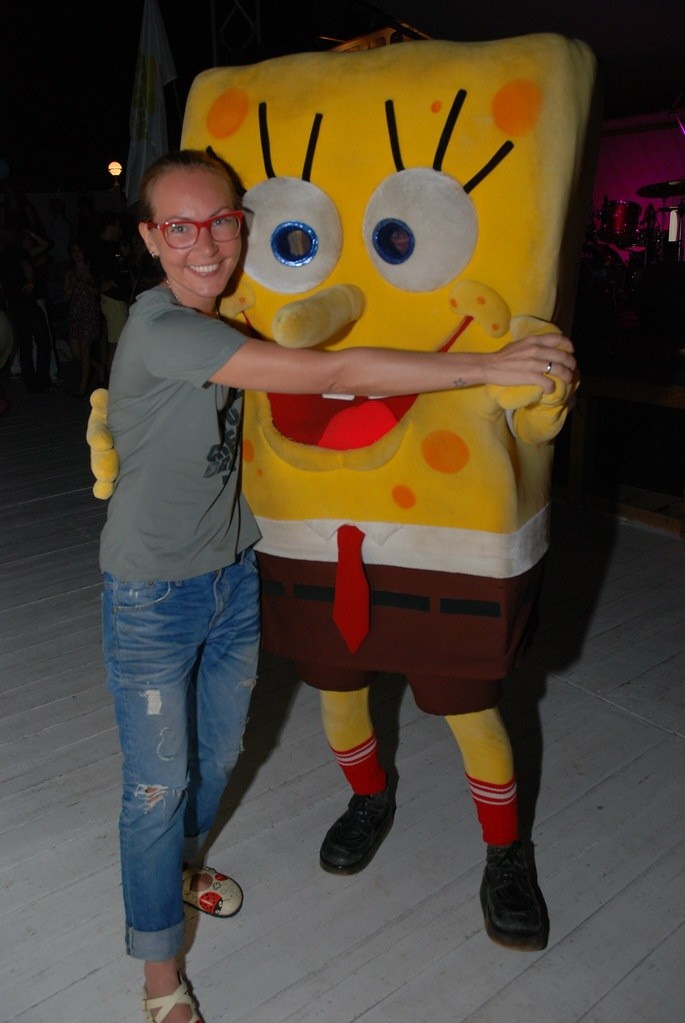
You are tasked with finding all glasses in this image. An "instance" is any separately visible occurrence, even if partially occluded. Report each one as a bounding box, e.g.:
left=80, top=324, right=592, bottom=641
left=147, top=209, right=247, bottom=249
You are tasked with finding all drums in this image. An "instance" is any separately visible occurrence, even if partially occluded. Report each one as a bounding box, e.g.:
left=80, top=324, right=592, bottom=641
left=591, top=244, right=647, bottom=299
left=601, top=199, right=642, bottom=239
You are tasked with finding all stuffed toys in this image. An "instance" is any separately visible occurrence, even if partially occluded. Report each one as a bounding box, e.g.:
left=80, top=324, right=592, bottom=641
left=83, top=34, right=598, bottom=953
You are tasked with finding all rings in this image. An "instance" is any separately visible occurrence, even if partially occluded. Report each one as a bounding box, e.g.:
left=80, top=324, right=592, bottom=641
left=546, top=361, right=552, bottom=374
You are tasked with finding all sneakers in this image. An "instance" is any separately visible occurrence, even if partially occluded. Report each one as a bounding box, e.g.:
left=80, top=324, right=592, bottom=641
left=319, top=779, right=397, bottom=876
left=479, top=839, right=550, bottom=951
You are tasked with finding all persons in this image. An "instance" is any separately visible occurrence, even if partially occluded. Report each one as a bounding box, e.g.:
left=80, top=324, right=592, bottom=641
left=98, top=147, right=578, bottom=1023
left=0, top=214, right=162, bottom=416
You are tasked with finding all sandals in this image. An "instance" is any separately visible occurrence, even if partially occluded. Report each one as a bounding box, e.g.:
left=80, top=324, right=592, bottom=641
left=141, top=974, right=203, bottom=1023
left=182, top=866, right=244, bottom=918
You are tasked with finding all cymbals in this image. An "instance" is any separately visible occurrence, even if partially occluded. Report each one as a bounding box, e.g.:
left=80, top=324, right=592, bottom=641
left=635, top=177, right=685, bottom=198
